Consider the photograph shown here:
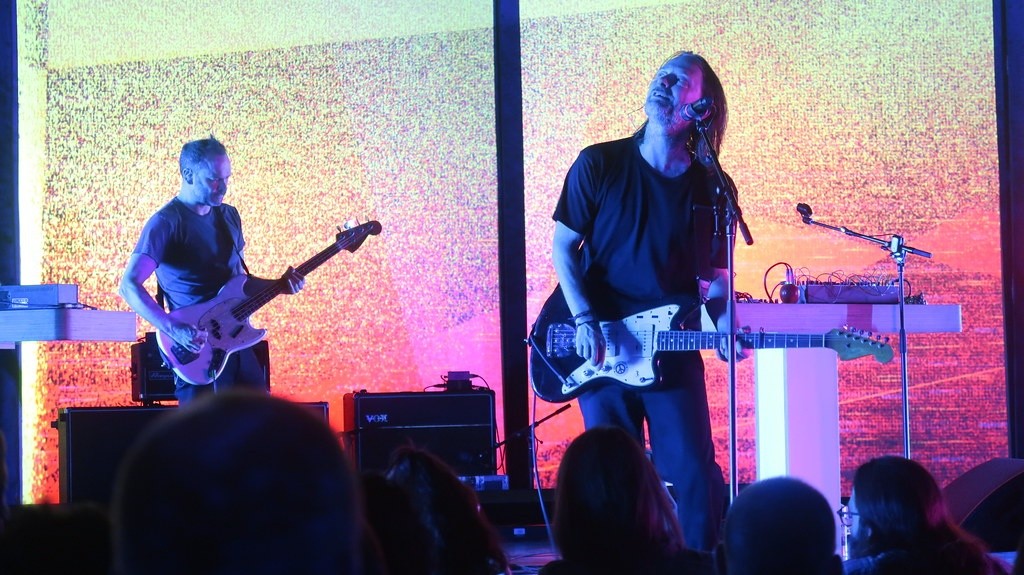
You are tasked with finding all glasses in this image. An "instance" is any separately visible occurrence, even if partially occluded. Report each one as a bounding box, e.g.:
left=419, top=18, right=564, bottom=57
left=838, top=506, right=861, bottom=527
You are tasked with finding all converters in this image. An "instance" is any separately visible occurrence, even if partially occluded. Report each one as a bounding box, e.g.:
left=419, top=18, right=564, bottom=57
left=446, top=371, right=473, bottom=392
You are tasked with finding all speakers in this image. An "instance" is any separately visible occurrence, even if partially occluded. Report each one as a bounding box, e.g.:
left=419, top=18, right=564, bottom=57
left=343, top=386, right=497, bottom=483
left=52, top=402, right=329, bottom=505
left=941, top=456, right=1024, bottom=552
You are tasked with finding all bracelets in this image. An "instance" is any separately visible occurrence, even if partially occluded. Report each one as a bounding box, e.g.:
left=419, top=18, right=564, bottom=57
left=573, top=310, right=599, bottom=327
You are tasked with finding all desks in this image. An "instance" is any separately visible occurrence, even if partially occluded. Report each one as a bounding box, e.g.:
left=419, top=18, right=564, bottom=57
left=0, top=311, right=136, bottom=343
left=737, top=303, right=962, bottom=558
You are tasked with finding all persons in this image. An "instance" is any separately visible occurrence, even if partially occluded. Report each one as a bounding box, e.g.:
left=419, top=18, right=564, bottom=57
left=551, top=51, right=754, bottom=553
left=119, top=138, right=304, bottom=410
left=0, top=394, right=1024, bottom=575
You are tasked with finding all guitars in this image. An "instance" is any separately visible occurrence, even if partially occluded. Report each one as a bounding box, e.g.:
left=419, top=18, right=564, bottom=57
left=527, top=280, right=897, bottom=404
left=155, top=214, right=383, bottom=386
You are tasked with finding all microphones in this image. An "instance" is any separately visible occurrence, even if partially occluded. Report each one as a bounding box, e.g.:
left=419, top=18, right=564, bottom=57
left=680, top=97, right=712, bottom=121
